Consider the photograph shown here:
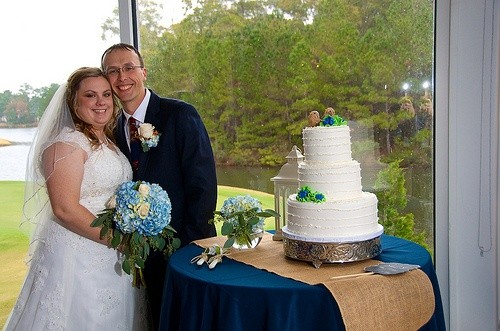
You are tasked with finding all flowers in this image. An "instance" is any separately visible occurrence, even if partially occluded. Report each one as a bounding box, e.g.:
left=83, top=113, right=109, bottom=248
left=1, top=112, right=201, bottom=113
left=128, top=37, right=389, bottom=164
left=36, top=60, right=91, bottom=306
left=204, top=243, right=222, bottom=258
left=208, top=195, right=282, bottom=249
left=134, top=122, right=160, bottom=152
left=90, top=180, right=181, bottom=289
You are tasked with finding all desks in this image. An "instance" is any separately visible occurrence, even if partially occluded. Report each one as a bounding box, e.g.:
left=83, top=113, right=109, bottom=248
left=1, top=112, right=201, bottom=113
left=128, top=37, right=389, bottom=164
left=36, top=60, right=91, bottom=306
left=158, top=229, right=446, bottom=331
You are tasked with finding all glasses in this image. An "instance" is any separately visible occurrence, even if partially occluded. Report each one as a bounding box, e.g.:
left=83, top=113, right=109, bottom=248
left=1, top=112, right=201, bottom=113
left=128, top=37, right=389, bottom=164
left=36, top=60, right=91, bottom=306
left=106, top=64, right=144, bottom=77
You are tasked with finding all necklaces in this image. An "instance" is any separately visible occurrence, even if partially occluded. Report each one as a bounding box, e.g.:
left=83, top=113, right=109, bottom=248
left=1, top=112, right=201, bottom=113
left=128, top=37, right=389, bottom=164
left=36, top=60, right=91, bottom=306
left=103, top=138, right=109, bottom=147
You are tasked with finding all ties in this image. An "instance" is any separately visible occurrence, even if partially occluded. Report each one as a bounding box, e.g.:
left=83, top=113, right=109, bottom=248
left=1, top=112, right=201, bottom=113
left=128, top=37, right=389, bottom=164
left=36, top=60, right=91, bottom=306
left=127, top=116, right=145, bottom=173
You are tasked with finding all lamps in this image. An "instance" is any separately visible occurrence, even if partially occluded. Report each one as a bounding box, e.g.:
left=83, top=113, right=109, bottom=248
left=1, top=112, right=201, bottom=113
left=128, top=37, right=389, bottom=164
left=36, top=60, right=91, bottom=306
left=270, top=145, right=305, bottom=240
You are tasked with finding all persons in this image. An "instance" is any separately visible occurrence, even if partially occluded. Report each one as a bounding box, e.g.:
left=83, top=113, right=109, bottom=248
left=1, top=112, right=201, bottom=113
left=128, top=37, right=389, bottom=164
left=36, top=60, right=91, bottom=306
left=100, top=43, right=218, bottom=331
left=4, top=66, right=148, bottom=331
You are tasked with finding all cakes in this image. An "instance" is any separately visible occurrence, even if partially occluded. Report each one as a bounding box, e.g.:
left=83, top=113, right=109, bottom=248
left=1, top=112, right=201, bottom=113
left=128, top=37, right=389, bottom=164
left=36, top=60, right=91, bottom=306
left=285, top=107, right=378, bottom=238
